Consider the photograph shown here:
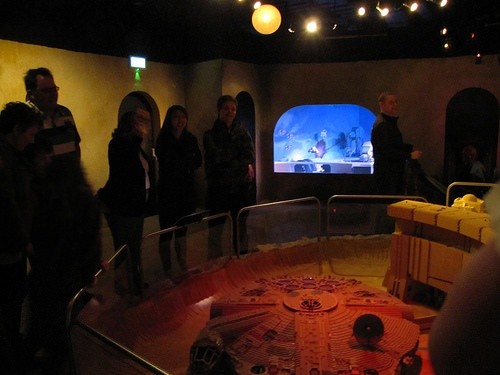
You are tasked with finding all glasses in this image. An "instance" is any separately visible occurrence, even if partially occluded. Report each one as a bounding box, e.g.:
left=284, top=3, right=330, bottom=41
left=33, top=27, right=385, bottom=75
left=35, top=87, right=59, bottom=92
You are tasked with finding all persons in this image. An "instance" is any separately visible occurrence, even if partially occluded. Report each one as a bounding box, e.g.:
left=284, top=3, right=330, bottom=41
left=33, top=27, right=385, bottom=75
left=371, top=92, right=423, bottom=235
left=429, top=183, right=500, bottom=375
left=0, top=67, right=197, bottom=368
left=202, top=94, right=254, bottom=260
